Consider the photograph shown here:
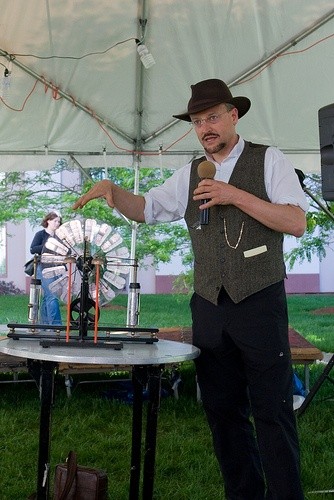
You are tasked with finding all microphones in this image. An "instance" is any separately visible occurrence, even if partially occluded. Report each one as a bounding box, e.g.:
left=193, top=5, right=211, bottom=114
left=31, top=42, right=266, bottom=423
left=197, top=160, right=216, bottom=226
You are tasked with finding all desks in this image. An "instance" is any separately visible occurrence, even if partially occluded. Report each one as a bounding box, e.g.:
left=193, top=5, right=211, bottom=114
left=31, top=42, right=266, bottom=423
left=0, top=336, right=201, bottom=500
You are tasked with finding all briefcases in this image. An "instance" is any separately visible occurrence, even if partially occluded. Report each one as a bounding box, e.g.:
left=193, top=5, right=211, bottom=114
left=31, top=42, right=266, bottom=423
left=54, top=451, right=107, bottom=500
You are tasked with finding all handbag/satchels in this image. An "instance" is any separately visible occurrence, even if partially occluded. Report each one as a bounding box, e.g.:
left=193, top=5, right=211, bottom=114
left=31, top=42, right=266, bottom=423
left=24, top=258, right=35, bottom=276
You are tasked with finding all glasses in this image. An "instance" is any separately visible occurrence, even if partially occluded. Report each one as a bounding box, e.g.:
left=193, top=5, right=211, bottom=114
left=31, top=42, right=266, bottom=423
left=189, top=111, right=231, bottom=129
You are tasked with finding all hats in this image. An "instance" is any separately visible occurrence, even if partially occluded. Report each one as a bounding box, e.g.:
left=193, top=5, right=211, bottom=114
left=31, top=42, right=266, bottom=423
left=171, top=79, right=250, bottom=122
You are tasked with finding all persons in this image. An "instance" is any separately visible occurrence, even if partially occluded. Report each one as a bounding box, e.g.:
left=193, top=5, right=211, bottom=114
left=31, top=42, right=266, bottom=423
left=28, top=212, right=65, bottom=333
left=69, top=79, right=311, bottom=499
